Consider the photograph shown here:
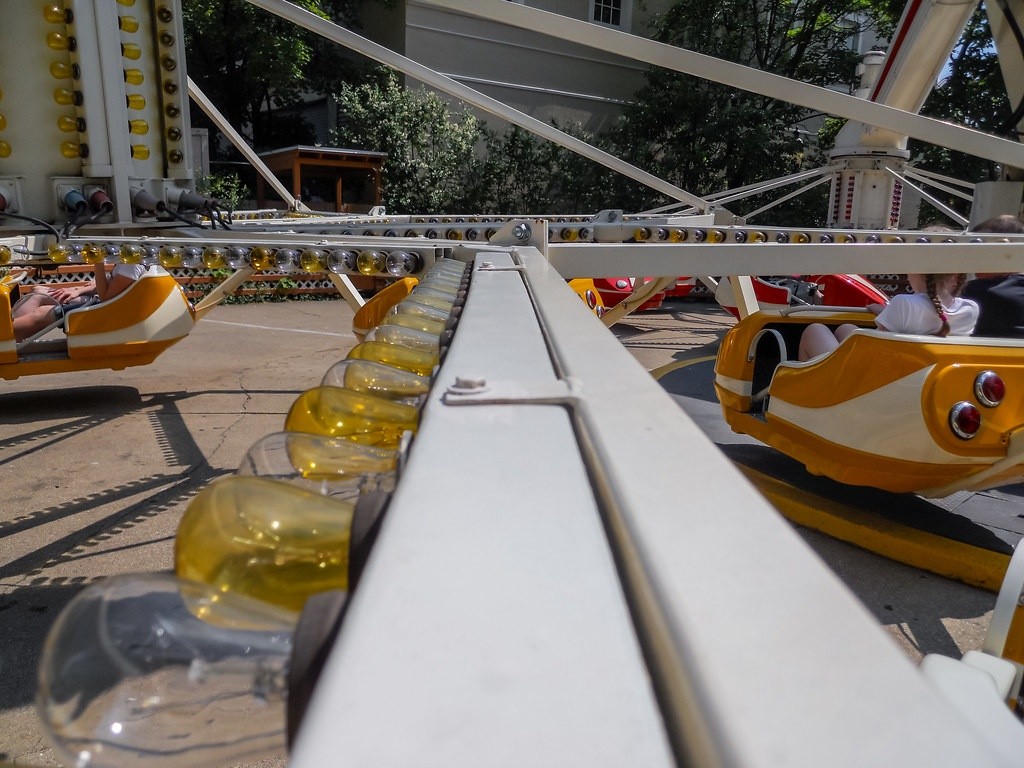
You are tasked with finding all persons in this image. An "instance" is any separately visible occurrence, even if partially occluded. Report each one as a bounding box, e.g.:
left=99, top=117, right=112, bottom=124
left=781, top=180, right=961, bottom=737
left=12, top=262, right=146, bottom=343
left=967, top=212, right=1023, bottom=338
left=798, top=224, right=983, bottom=372
